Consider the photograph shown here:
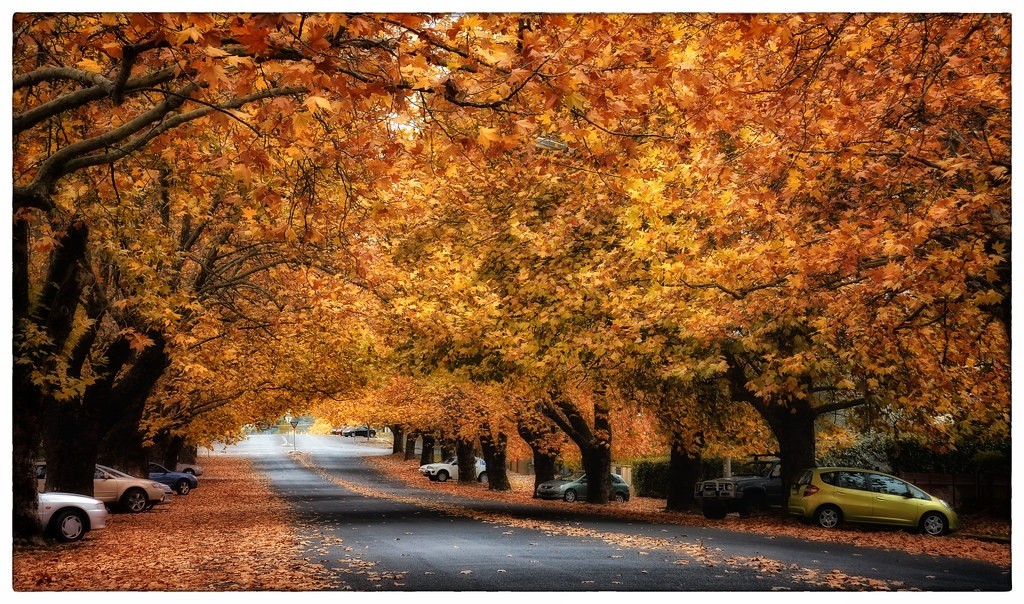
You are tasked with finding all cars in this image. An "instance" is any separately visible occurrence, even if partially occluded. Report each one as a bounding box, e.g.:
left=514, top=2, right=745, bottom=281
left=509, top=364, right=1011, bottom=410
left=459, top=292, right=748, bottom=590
left=536, top=470, right=629, bottom=503
left=788, top=467, right=958, bottom=537
left=148, top=462, right=199, bottom=496
left=419, top=456, right=489, bottom=483
left=330, top=425, right=349, bottom=436
left=342, top=426, right=376, bottom=438
left=38, top=491, right=108, bottom=541
left=36, top=462, right=173, bottom=513
left=176, top=463, right=203, bottom=477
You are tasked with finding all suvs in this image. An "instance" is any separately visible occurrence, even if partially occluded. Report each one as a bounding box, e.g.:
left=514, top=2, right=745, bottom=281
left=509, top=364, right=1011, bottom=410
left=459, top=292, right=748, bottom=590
left=695, top=457, right=786, bottom=519
